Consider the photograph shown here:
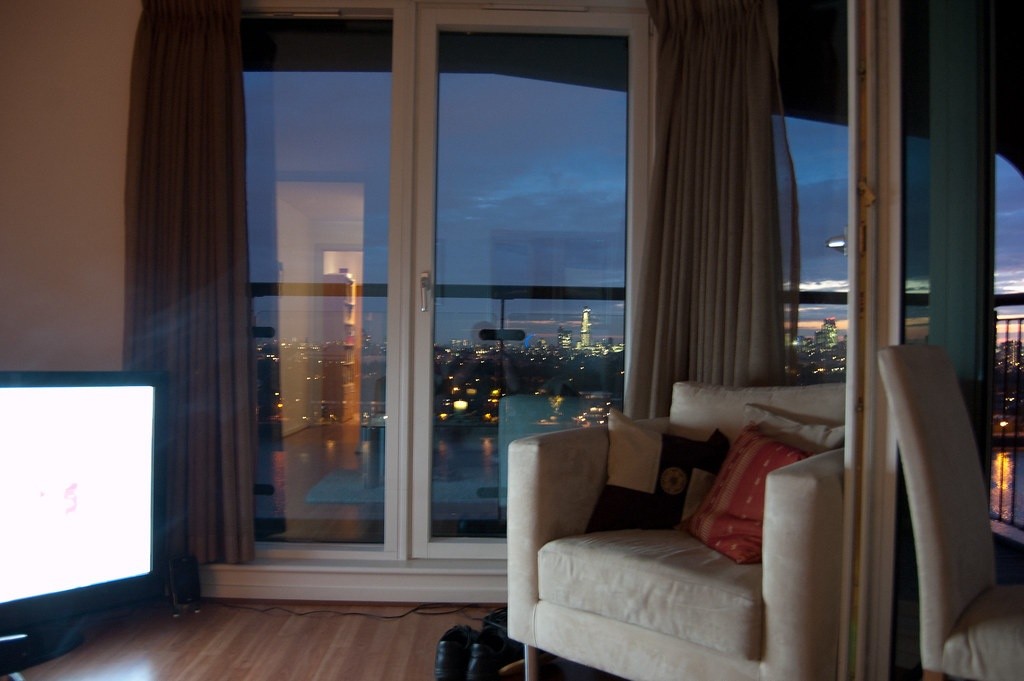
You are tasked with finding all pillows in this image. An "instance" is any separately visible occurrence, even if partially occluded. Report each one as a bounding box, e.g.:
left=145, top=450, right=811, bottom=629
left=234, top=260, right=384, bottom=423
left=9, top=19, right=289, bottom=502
left=738, top=401, right=846, bottom=459
left=587, top=405, right=730, bottom=534
left=686, top=423, right=812, bottom=564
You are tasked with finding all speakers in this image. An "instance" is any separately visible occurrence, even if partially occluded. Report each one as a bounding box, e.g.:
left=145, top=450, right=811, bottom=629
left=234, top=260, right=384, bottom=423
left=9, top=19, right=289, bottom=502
left=170, top=555, right=201, bottom=604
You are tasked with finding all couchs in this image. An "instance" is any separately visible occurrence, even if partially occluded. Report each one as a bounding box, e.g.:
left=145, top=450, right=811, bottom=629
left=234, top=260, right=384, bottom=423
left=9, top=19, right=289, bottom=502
left=501, top=383, right=845, bottom=681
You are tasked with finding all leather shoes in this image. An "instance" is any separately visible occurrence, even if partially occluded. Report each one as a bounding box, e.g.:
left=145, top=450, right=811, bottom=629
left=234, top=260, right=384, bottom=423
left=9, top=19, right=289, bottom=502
left=431, top=622, right=525, bottom=681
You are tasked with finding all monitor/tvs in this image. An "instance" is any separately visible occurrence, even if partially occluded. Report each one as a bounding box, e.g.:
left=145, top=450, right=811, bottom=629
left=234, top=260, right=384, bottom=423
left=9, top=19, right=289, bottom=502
left=0, top=369, right=167, bottom=631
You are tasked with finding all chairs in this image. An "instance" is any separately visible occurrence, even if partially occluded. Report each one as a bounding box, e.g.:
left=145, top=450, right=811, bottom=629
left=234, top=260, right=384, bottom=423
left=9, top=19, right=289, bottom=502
left=876, top=340, right=1024, bottom=679
